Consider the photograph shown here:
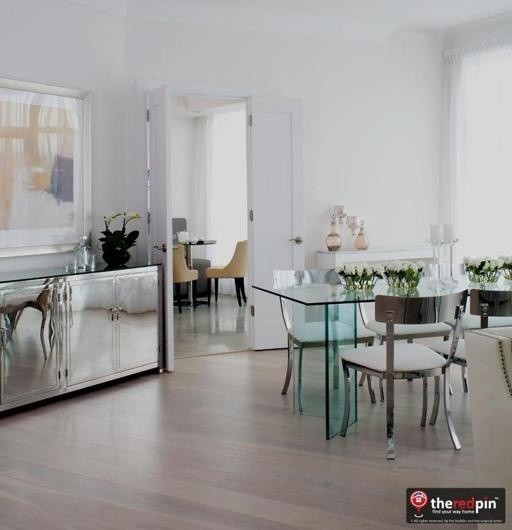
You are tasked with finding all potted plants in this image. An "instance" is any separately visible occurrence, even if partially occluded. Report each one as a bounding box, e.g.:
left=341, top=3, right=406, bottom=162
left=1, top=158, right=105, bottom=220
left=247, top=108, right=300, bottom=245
left=94, top=210, right=144, bottom=269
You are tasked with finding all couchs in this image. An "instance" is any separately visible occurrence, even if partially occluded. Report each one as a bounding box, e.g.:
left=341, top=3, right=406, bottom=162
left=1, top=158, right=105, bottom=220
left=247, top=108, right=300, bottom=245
left=467, top=326, right=511, bottom=530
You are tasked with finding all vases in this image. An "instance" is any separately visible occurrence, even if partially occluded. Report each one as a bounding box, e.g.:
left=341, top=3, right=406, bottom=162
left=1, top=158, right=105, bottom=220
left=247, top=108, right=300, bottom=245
left=345, top=275, right=377, bottom=291
left=388, top=275, right=420, bottom=287
left=471, top=273, right=500, bottom=284
left=102, top=251, right=131, bottom=269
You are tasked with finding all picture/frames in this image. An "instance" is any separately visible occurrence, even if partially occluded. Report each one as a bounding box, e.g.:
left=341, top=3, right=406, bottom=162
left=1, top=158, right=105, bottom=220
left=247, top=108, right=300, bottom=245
left=1, top=76, right=93, bottom=259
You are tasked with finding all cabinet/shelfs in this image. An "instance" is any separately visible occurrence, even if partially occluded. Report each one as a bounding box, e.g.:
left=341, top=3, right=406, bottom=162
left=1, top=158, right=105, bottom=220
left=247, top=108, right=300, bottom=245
left=69, top=272, right=158, bottom=391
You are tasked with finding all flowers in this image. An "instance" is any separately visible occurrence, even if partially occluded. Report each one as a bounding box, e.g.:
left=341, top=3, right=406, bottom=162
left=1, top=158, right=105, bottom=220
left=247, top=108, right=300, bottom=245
left=461, top=257, right=503, bottom=283
left=380, top=263, right=425, bottom=286
left=334, top=262, right=382, bottom=289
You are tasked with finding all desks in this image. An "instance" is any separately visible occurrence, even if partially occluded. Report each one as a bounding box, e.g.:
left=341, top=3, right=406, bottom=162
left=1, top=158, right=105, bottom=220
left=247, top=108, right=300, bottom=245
left=252, top=273, right=509, bottom=441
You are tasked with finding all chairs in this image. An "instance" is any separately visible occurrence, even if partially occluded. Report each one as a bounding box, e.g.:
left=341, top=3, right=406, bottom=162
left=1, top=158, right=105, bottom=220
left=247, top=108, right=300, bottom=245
left=337, top=292, right=462, bottom=460
left=355, top=258, right=455, bottom=428
left=471, top=285, right=512, bottom=324
left=205, top=239, right=248, bottom=308
left=273, top=267, right=353, bottom=416
left=173, top=245, right=200, bottom=315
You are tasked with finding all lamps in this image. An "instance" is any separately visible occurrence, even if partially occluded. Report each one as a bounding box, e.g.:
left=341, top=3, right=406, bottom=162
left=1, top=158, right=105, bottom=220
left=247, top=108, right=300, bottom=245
left=427, top=222, right=444, bottom=280
left=445, top=222, right=461, bottom=277
